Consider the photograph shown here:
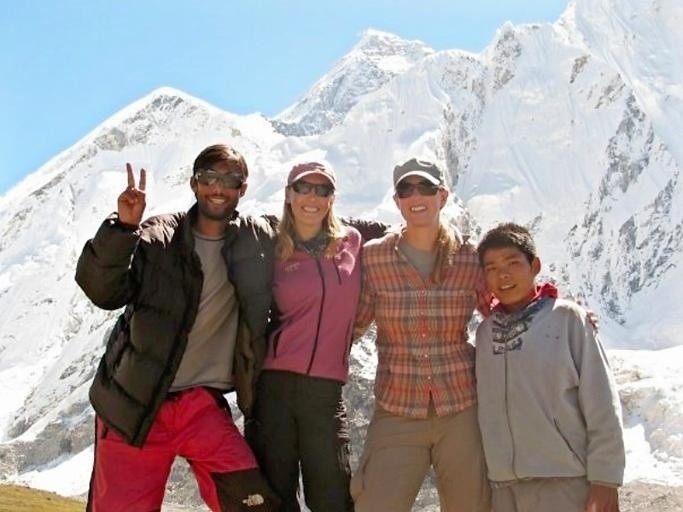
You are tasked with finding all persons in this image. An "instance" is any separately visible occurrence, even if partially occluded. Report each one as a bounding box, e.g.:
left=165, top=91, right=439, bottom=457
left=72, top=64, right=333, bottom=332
left=348, top=156, right=599, bottom=512
left=73, top=144, right=404, bottom=512
left=242, top=161, right=364, bottom=512
left=472, top=222, right=625, bottom=512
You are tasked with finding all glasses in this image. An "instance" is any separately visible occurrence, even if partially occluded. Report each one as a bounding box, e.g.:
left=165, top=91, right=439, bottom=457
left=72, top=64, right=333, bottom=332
left=396, top=180, right=438, bottom=198
left=194, top=169, right=243, bottom=190
left=292, top=179, right=332, bottom=196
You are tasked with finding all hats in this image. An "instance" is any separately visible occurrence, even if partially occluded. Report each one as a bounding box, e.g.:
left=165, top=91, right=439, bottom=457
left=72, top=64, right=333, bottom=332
left=288, top=163, right=336, bottom=189
left=393, top=159, right=444, bottom=189
